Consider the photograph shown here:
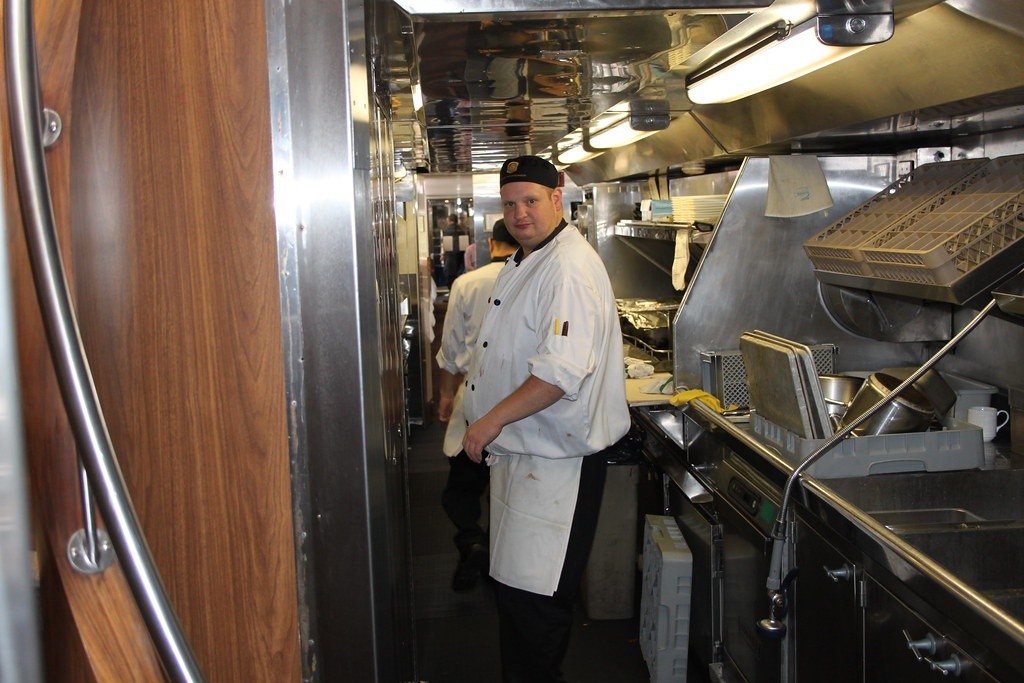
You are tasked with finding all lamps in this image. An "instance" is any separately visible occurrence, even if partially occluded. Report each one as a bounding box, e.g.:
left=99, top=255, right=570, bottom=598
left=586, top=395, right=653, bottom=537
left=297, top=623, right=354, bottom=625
left=548, top=114, right=671, bottom=173
left=685, top=0, right=894, bottom=106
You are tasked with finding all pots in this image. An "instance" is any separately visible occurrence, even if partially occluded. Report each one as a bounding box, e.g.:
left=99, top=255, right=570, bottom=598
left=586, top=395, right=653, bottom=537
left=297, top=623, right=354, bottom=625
left=818, top=367, right=957, bottom=436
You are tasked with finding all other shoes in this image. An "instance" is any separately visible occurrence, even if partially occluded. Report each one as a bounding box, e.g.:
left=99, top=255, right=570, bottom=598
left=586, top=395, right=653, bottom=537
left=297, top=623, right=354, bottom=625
left=451, top=543, right=489, bottom=594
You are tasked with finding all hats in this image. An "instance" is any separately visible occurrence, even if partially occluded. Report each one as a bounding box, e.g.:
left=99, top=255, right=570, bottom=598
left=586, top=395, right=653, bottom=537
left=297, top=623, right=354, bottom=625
left=493, top=219, right=520, bottom=246
left=500, top=155, right=558, bottom=189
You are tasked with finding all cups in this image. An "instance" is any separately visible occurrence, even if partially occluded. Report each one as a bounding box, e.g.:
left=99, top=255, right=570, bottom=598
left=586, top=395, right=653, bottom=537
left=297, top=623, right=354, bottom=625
left=968, top=406, right=1009, bottom=443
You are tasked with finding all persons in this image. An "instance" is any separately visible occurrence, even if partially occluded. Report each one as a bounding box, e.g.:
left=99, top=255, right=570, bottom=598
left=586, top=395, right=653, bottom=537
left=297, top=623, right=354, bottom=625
left=462, top=155, right=631, bottom=683
left=432, top=204, right=523, bottom=593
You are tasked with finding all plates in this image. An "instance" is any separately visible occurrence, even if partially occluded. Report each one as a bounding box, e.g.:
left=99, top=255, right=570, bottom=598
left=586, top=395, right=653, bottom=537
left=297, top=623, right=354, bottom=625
left=671, top=194, right=728, bottom=225
